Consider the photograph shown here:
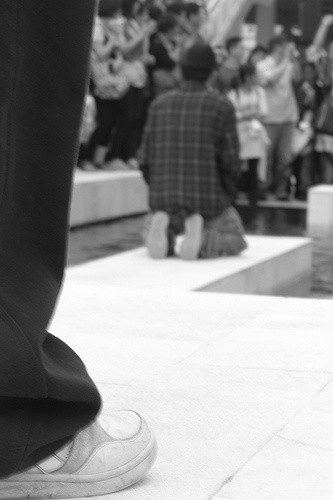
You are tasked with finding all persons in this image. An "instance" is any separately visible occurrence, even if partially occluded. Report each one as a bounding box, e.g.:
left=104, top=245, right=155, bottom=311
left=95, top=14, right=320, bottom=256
left=81, top=0, right=333, bottom=200
left=0, top=0, right=155, bottom=499
left=137, top=37, right=247, bottom=259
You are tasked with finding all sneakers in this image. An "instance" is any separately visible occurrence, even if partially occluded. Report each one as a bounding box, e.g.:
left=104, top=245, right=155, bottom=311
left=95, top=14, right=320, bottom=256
left=0, top=408, right=155, bottom=499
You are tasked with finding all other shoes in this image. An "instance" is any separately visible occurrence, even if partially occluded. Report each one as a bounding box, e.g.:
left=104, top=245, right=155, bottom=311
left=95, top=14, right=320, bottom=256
left=148, top=210, right=175, bottom=257
left=178, top=212, right=205, bottom=260
